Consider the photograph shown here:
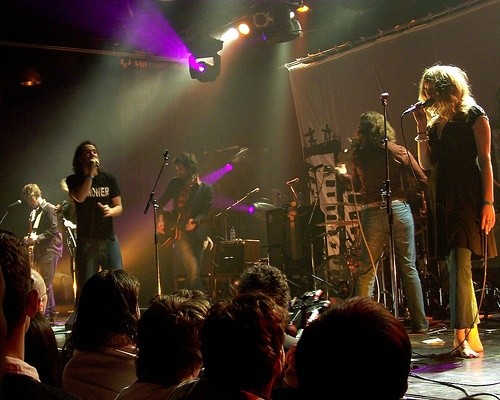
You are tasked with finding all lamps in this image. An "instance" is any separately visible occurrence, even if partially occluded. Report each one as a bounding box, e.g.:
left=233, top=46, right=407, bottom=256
left=186, top=32, right=225, bottom=83
left=252, top=0, right=304, bottom=44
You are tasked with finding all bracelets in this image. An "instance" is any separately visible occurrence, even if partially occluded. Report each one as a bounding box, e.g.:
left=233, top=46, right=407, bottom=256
left=86, top=174, right=95, bottom=179
left=415, top=136, right=429, bottom=142
left=416, top=132, right=428, bottom=134
left=484, top=201, right=494, bottom=205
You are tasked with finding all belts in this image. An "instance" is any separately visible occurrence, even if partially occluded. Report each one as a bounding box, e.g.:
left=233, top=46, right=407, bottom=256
left=361, top=197, right=407, bottom=208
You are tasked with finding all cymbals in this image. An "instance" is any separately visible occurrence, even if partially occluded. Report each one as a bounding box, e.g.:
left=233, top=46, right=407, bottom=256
left=315, top=221, right=361, bottom=226
left=324, top=202, right=364, bottom=207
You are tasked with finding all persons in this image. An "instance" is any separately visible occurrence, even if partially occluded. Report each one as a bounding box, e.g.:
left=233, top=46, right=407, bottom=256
left=66, top=141, right=123, bottom=308
left=351, top=111, right=428, bottom=332
left=157, top=158, right=212, bottom=291
left=0, top=231, right=412, bottom=400
left=43, top=231, right=62, bottom=316
left=411, top=64, right=495, bottom=359
left=21, top=184, right=58, bottom=287
left=54, top=193, right=77, bottom=279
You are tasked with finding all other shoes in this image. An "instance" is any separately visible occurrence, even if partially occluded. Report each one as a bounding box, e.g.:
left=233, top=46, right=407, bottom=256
left=44, top=307, right=55, bottom=317
left=412, top=325, right=428, bottom=333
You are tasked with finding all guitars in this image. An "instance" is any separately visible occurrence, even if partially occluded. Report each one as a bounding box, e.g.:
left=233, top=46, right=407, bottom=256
left=158, top=209, right=225, bottom=248
left=28, top=209, right=37, bottom=270
left=285, top=201, right=313, bottom=302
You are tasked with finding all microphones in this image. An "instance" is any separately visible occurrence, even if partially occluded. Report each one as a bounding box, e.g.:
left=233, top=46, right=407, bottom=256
left=247, top=187, right=260, bottom=196
left=403, top=98, right=433, bottom=115
left=310, top=164, right=323, bottom=171
left=7, top=199, right=22, bottom=207
left=285, top=177, right=299, bottom=185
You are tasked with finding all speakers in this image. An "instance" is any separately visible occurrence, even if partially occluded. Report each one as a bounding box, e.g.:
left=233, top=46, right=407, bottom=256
left=216, top=239, right=260, bottom=273
left=266, top=205, right=326, bottom=299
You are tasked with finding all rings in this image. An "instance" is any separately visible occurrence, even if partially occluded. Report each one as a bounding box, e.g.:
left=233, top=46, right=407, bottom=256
left=485, top=219, right=490, bottom=224
